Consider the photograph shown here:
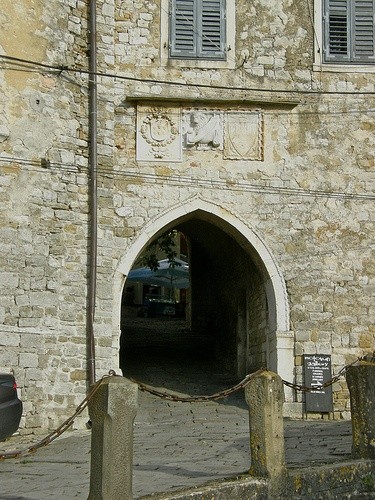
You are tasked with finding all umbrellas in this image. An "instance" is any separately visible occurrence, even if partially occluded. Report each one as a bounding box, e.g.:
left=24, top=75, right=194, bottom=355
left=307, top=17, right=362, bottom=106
left=126, top=256, right=191, bottom=302
